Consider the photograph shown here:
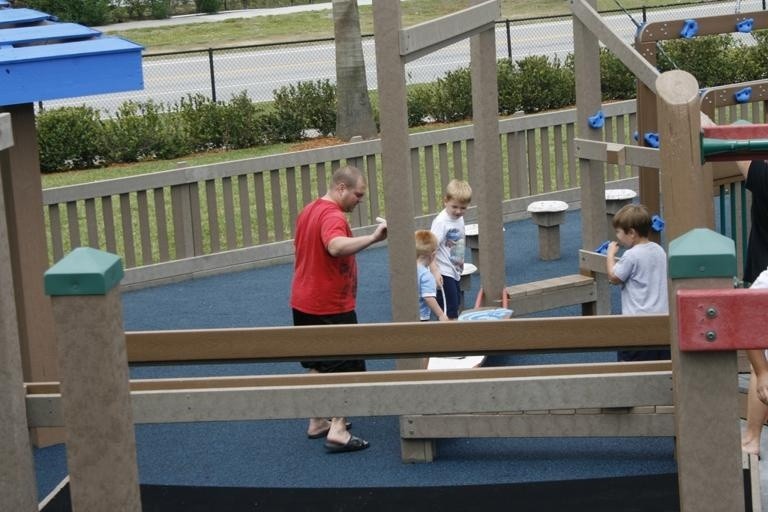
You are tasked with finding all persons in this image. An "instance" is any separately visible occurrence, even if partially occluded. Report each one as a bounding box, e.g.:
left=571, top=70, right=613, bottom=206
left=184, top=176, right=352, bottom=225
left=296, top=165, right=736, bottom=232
left=414, top=229, right=450, bottom=323
left=430, top=179, right=472, bottom=323
left=290, top=165, right=389, bottom=452
left=606, top=204, right=666, bottom=362
left=743, top=160, right=768, bottom=456
left=742, top=269, right=768, bottom=405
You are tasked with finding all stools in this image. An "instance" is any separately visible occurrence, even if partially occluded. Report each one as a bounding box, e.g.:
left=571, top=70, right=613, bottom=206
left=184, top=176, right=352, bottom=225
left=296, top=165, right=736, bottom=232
left=524, top=197, right=572, bottom=265
left=463, top=220, right=509, bottom=270
left=604, top=184, right=639, bottom=242
left=457, top=262, right=479, bottom=306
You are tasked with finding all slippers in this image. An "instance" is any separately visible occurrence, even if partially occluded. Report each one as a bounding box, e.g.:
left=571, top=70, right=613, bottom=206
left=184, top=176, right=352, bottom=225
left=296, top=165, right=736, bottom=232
left=300, top=420, right=353, bottom=438
left=321, top=433, right=371, bottom=456
